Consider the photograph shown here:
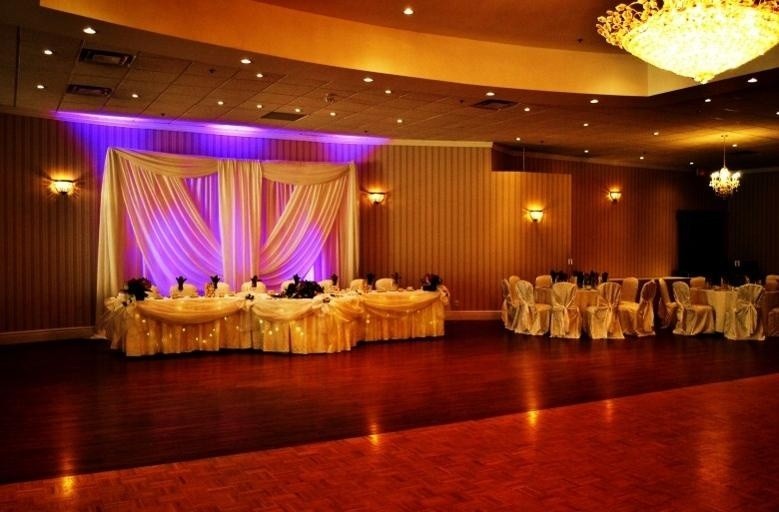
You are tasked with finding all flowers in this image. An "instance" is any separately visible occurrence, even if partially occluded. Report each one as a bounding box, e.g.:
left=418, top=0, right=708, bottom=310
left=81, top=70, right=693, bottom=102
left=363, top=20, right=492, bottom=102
left=419, top=273, right=443, bottom=285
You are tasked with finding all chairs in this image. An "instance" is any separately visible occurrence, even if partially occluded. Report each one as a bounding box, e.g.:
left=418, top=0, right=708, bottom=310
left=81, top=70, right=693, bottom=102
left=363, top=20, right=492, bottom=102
left=501, top=268, right=779, bottom=342
left=109, top=273, right=404, bottom=297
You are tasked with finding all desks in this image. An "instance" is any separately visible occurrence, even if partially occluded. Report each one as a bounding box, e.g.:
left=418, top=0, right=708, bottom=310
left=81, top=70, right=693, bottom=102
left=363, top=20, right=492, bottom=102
left=102, top=285, right=449, bottom=359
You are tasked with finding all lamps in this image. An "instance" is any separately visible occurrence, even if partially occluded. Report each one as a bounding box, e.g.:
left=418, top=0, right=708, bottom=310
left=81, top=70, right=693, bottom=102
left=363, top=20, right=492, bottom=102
left=609, top=191, right=622, bottom=202
left=367, top=192, right=385, bottom=206
left=592, top=0, right=779, bottom=86
left=528, top=210, right=543, bottom=224
left=709, top=134, right=743, bottom=201
left=53, top=179, right=74, bottom=196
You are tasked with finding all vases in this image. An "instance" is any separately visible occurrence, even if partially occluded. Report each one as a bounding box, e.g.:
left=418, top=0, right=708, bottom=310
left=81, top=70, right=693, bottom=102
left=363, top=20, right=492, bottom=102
left=422, top=285, right=438, bottom=290
